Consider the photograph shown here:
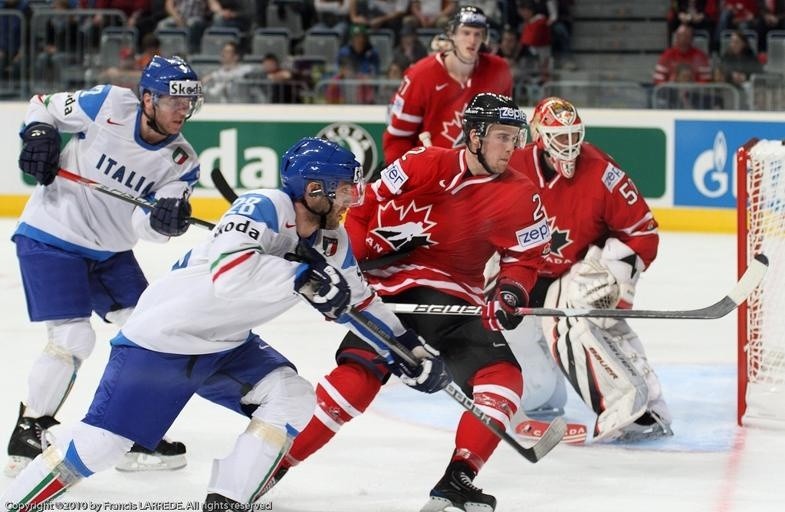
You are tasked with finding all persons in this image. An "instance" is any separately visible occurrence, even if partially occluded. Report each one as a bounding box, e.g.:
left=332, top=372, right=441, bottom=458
left=379, top=3, right=512, bottom=164
left=248, top=82, right=555, bottom=511
left=4, top=51, right=203, bottom=464
left=504, top=95, right=674, bottom=444
left=645, top=1, right=784, bottom=109
left=3, top=0, right=567, bottom=107
left=0, top=136, right=450, bottom=509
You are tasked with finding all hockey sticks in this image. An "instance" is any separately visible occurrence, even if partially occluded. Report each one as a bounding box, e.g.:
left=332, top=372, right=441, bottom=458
left=383, top=252, right=770, bottom=320
left=210, top=168, right=239, bottom=204
left=309, top=275, right=568, bottom=464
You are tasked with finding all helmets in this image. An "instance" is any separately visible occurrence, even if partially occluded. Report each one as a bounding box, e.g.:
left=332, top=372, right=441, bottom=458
left=453, top=6, right=488, bottom=38
left=463, top=93, right=585, bottom=179
left=138, top=54, right=204, bottom=114
left=280, top=137, right=365, bottom=208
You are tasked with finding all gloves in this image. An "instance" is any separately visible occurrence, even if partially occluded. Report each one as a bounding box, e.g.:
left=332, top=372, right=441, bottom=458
left=150, top=199, right=191, bottom=236
left=567, top=238, right=645, bottom=309
left=383, top=328, right=453, bottom=393
left=19, top=122, right=60, bottom=186
left=482, top=277, right=530, bottom=331
left=294, top=264, right=350, bottom=319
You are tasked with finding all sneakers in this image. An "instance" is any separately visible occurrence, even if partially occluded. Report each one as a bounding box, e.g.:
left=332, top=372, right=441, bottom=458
left=8, top=402, right=60, bottom=459
left=430, top=461, right=496, bottom=512
left=129, top=438, right=186, bottom=456
